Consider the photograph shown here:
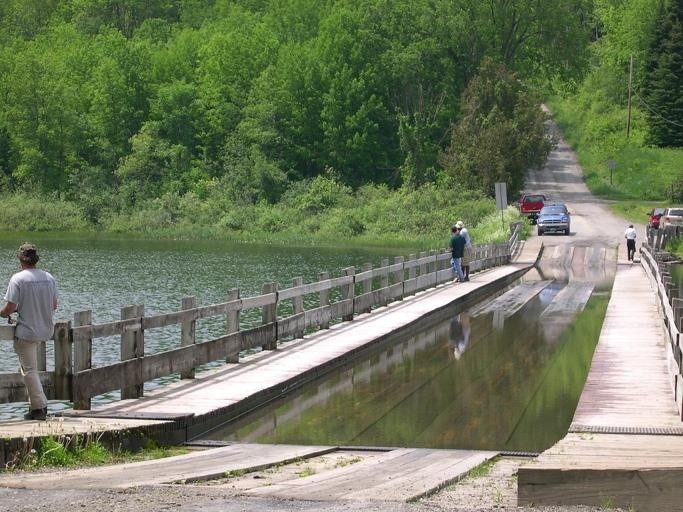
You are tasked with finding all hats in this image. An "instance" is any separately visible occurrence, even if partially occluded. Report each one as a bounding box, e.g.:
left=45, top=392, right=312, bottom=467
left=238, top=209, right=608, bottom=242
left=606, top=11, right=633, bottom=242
left=17, top=242, right=39, bottom=264
left=455, top=221, right=463, bottom=228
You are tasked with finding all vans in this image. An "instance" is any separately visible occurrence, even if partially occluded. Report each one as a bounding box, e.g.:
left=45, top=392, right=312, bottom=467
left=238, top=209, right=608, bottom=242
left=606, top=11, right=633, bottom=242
left=520, top=193, right=546, bottom=217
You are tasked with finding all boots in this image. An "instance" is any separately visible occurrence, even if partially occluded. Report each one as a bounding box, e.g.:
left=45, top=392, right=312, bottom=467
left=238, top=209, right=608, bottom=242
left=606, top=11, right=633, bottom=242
left=464, top=267, right=469, bottom=281
left=461, top=266, right=464, bottom=275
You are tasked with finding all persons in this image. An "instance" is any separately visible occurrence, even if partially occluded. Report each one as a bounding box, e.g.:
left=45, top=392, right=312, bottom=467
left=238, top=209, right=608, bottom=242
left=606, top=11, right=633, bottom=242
left=454, top=309, right=471, bottom=361
left=0, top=241, right=58, bottom=421
left=448, top=313, right=465, bottom=349
left=455, top=220, right=471, bottom=281
left=624, top=223, right=637, bottom=262
left=447, top=225, right=465, bottom=282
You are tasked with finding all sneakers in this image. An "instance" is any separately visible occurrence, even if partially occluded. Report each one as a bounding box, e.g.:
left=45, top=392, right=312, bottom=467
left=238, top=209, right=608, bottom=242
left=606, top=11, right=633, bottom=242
left=457, top=279, right=463, bottom=281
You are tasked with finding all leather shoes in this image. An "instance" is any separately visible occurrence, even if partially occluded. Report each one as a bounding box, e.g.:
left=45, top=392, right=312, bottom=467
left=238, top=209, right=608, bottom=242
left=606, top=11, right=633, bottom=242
left=24, top=409, right=46, bottom=420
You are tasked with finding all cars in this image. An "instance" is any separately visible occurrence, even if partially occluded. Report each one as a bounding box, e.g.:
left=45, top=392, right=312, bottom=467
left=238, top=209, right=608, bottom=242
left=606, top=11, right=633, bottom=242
left=647, top=207, right=683, bottom=231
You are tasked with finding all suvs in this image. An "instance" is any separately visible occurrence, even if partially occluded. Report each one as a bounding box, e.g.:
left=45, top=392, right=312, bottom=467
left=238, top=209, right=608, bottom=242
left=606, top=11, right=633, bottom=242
left=536, top=205, right=570, bottom=235
